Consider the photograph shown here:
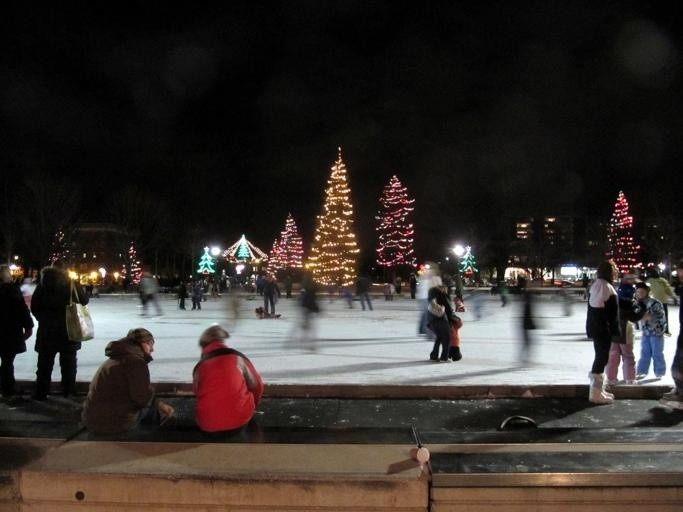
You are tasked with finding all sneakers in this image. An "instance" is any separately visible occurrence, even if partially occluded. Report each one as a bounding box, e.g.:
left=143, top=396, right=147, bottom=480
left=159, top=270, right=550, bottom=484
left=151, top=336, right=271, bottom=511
left=658, top=388, right=683, bottom=401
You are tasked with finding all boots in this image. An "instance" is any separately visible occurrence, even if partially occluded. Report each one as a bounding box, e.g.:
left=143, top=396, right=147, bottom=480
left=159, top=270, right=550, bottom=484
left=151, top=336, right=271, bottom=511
left=588, top=372, right=614, bottom=404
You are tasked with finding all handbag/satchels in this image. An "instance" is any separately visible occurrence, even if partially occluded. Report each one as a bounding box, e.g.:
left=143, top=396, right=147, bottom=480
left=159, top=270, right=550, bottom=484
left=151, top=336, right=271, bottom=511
left=64, top=276, right=95, bottom=342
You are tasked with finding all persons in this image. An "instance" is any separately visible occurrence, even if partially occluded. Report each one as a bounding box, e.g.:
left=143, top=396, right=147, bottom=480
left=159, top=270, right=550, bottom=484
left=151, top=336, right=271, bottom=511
left=587, top=261, right=619, bottom=405
left=2, top=265, right=33, bottom=409
left=31, top=259, right=92, bottom=398
left=427, top=285, right=452, bottom=362
left=606, top=283, right=647, bottom=386
left=410, top=273, right=417, bottom=299
left=663, top=261, right=682, bottom=402
left=636, top=282, right=667, bottom=379
left=190, top=280, right=201, bottom=310
left=80, top=328, right=174, bottom=443
left=284, top=276, right=292, bottom=298
left=139, top=267, right=162, bottom=316
left=646, top=269, right=680, bottom=336
left=178, top=280, right=189, bottom=311
left=356, top=274, right=372, bottom=311
left=260, top=274, right=280, bottom=315
left=449, top=308, right=462, bottom=361
left=192, top=326, right=264, bottom=443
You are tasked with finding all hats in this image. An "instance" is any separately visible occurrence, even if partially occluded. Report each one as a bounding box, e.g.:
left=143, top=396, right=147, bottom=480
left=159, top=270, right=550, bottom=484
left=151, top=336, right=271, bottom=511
left=127, top=328, right=155, bottom=347
left=199, top=326, right=231, bottom=348
